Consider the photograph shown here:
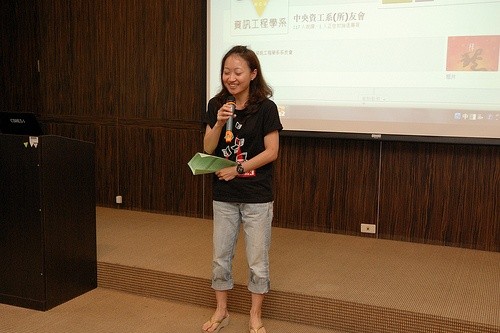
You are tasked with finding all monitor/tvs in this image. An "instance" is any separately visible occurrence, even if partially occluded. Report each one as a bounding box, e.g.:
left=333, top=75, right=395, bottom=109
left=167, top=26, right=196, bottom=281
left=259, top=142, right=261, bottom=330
left=0, top=111, right=44, bottom=136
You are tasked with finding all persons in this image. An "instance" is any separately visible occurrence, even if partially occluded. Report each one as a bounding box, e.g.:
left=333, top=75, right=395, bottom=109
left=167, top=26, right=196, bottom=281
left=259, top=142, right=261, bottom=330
left=201, top=45, right=279, bottom=333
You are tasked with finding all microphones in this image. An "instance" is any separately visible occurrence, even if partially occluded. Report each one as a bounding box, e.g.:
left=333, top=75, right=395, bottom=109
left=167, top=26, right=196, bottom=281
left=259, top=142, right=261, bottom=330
left=225, top=96, right=237, bottom=143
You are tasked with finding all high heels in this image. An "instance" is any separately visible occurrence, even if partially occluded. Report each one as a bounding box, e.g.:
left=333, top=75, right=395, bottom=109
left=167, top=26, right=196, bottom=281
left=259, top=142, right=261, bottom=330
left=203, top=313, right=230, bottom=333
left=248, top=320, right=266, bottom=333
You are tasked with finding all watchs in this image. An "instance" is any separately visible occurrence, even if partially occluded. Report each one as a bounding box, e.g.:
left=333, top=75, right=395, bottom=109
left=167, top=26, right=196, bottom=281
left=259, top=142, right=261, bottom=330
left=237, top=163, right=245, bottom=174
